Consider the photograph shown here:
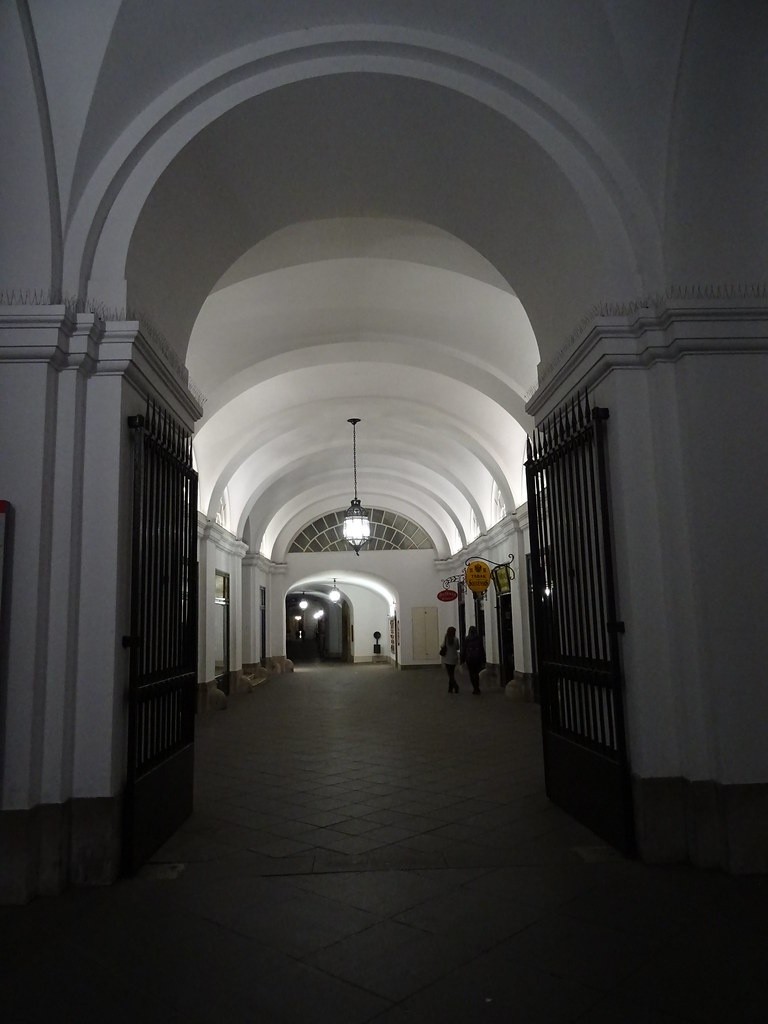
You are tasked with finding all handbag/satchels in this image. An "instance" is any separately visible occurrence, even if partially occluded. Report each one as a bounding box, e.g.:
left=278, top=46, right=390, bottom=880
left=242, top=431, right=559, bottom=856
left=439, top=636, right=448, bottom=657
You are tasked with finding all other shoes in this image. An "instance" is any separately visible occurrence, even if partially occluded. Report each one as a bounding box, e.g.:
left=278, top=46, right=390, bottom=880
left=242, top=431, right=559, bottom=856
left=448, top=683, right=453, bottom=693
left=453, top=685, right=459, bottom=693
left=472, top=689, right=480, bottom=695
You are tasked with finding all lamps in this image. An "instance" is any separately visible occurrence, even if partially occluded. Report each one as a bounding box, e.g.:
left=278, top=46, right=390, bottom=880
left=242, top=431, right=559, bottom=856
left=329, top=577, right=340, bottom=606
left=343, top=418, right=371, bottom=556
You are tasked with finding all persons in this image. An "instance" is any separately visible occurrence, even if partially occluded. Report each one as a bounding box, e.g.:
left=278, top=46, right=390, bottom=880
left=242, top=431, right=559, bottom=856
left=439, top=626, right=460, bottom=695
left=295, top=622, right=305, bottom=640
left=315, top=624, right=326, bottom=655
left=461, top=626, right=487, bottom=696
